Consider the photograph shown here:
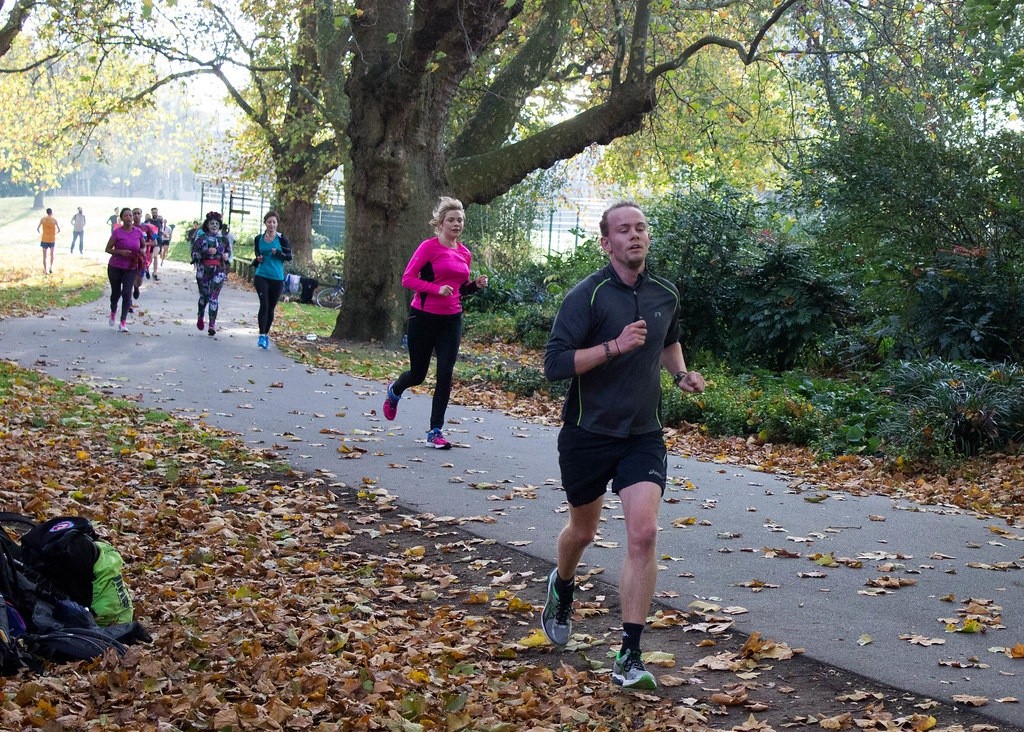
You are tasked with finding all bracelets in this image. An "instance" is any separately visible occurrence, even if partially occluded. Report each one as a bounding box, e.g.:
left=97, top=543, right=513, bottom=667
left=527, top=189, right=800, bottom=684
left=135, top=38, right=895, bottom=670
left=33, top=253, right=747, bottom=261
left=674, top=371, right=689, bottom=386
left=603, top=342, right=614, bottom=362
left=614, top=338, right=622, bottom=355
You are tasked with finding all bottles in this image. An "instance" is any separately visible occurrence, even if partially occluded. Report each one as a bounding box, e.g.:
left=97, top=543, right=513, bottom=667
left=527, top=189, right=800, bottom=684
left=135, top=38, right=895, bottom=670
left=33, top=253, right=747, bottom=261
left=402, top=335, right=407, bottom=345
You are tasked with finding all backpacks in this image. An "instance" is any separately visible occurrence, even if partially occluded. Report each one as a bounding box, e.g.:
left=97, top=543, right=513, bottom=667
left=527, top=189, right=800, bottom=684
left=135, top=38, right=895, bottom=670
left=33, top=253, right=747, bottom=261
left=0, top=510, right=134, bottom=676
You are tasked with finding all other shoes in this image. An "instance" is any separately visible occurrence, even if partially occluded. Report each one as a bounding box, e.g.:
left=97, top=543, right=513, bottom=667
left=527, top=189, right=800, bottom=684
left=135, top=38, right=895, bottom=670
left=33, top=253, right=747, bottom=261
left=152, top=272, right=159, bottom=282
left=146, top=271, right=150, bottom=278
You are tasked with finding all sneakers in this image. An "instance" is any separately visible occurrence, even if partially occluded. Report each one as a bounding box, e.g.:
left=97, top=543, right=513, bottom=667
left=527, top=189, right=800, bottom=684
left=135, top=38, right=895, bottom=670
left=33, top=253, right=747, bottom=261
left=109, top=311, right=116, bottom=325
left=382, top=381, right=401, bottom=420
left=258, top=334, right=269, bottom=351
left=612, top=652, right=657, bottom=691
left=197, top=316, right=205, bottom=331
left=133, top=286, right=139, bottom=299
left=426, top=428, right=452, bottom=449
left=129, top=307, right=134, bottom=314
left=541, top=564, right=576, bottom=647
left=207, top=327, right=215, bottom=337
left=119, top=324, right=129, bottom=332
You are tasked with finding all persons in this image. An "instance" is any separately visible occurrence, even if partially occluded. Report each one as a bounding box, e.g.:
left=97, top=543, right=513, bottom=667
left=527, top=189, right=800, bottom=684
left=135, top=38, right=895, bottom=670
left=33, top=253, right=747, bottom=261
left=37, top=208, right=60, bottom=275
left=70, top=208, right=86, bottom=254
left=539, top=202, right=706, bottom=690
left=383, top=197, right=488, bottom=449
left=187, top=212, right=234, bottom=336
left=104, top=206, right=174, bottom=333
left=249, top=211, right=293, bottom=351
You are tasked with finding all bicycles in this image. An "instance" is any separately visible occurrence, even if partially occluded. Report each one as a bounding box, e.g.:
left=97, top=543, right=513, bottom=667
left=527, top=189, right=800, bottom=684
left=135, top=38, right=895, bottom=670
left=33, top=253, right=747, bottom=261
left=315, top=274, right=345, bottom=310
